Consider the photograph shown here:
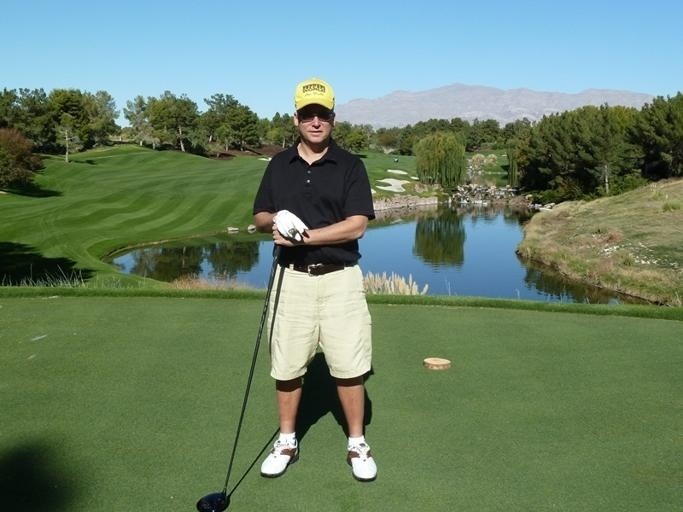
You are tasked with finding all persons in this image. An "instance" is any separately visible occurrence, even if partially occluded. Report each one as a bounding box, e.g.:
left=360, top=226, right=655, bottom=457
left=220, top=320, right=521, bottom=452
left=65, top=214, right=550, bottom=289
left=251, top=76, right=379, bottom=484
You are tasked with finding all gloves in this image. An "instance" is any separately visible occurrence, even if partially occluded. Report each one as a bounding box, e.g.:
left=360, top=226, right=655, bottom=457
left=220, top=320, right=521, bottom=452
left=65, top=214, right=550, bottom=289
left=272, top=209, right=309, bottom=242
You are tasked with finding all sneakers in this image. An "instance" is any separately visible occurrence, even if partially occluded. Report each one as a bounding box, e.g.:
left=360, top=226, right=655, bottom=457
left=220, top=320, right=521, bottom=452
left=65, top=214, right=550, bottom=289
left=261, top=439, right=299, bottom=478
left=346, top=441, right=377, bottom=482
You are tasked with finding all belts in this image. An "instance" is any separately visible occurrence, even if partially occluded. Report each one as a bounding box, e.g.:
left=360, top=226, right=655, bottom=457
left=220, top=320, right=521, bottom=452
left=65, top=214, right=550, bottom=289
left=276, top=257, right=358, bottom=277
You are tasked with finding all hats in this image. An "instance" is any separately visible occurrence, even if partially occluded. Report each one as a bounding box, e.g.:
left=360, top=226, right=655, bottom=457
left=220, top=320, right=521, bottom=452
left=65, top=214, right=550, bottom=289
left=294, top=79, right=336, bottom=112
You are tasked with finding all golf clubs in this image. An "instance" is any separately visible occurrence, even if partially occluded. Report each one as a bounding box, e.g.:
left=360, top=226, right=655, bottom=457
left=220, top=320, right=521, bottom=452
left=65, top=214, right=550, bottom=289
left=195, top=245, right=280, bottom=512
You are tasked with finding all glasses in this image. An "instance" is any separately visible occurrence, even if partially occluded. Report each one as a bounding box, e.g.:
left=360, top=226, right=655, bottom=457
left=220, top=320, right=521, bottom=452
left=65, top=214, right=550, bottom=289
left=297, top=110, right=334, bottom=122
